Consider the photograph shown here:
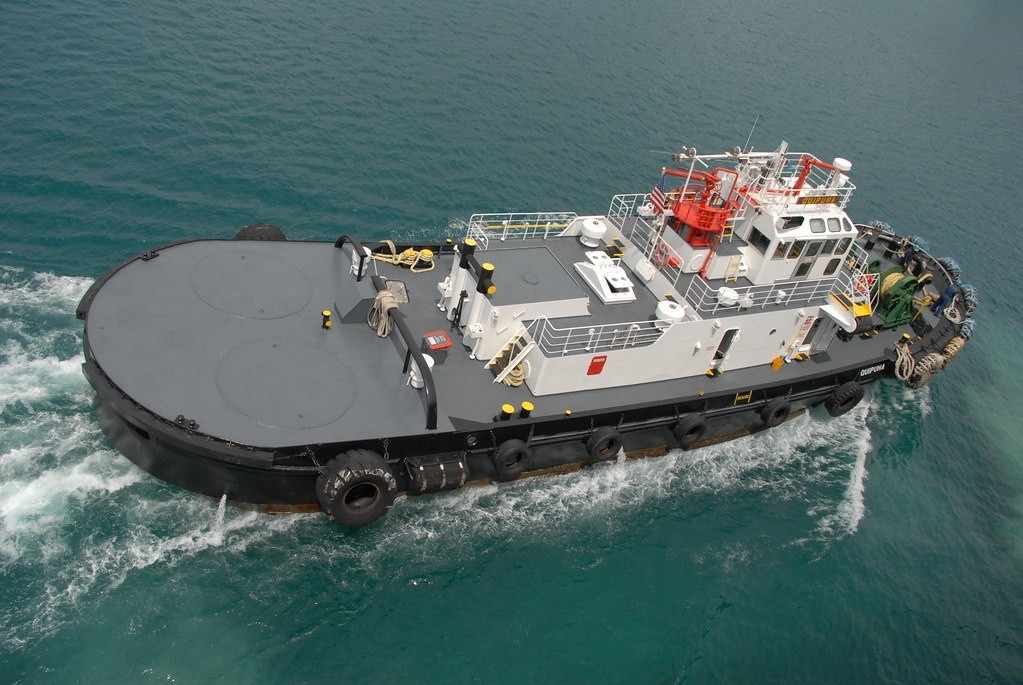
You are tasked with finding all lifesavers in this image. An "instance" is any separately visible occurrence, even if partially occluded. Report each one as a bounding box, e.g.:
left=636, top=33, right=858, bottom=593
left=825, top=381, right=864, bottom=416
left=673, top=415, right=707, bottom=446
left=315, top=450, right=397, bottom=526
left=235, top=223, right=287, bottom=240
left=857, top=275, right=872, bottom=291
left=656, top=242, right=670, bottom=266
left=761, top=397, right=790, bottom=427
left=586, top=426, right=623, bottom=462
left=491, top=439, right=532, bottom=477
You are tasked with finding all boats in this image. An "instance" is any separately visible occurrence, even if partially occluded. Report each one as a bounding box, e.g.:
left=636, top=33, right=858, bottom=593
left=72, top=114, right=980, bottom=529
